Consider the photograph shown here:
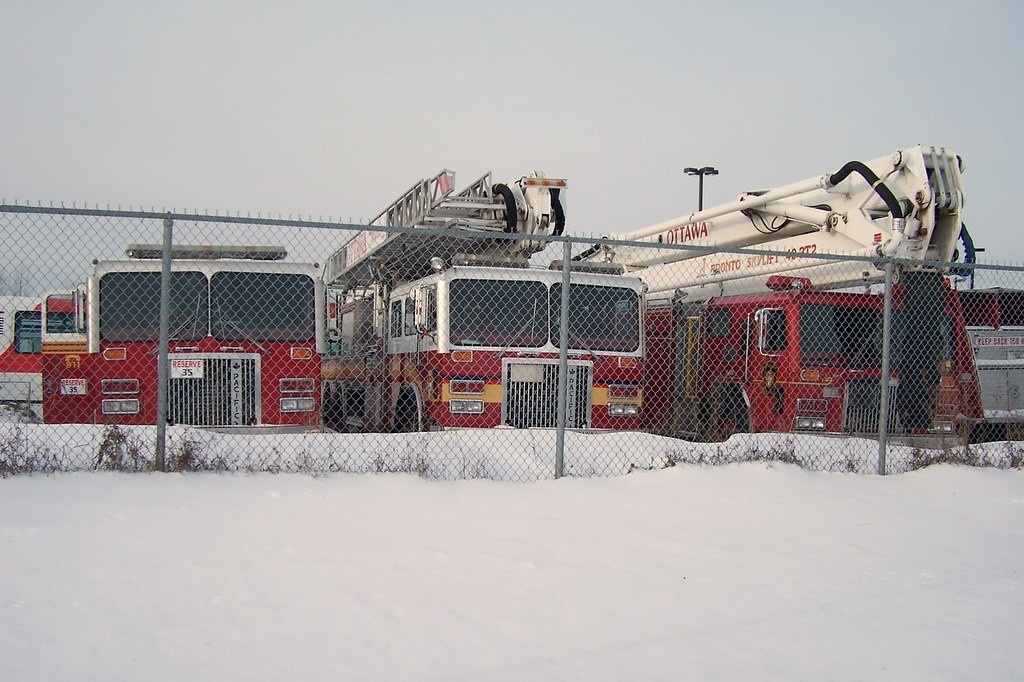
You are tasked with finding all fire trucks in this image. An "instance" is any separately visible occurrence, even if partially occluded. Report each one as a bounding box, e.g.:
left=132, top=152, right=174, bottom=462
left=0, top=243, right=325, bottom=435
left=322, top=170, right=648, bottom=431
left=574, top=141, right=985, bottom=447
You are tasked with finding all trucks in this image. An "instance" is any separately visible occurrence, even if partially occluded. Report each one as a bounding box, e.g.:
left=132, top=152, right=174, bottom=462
left=959, top=287, right=1024, bottom=424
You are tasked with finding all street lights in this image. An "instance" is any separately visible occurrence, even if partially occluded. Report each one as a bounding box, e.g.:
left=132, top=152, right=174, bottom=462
left=683, top=165, right=719, bottom=212
